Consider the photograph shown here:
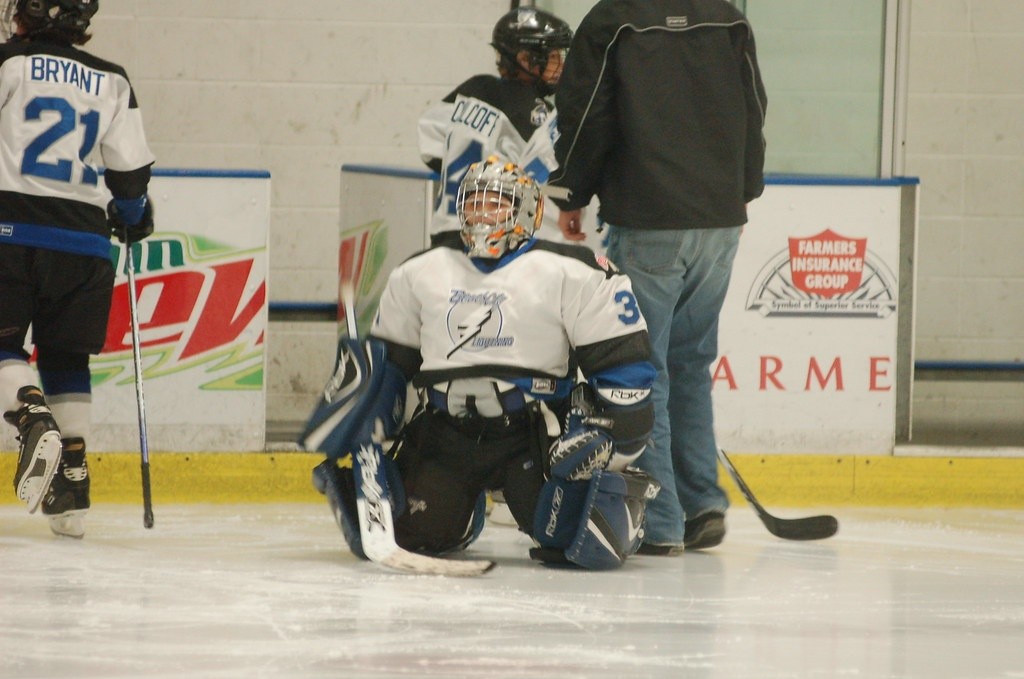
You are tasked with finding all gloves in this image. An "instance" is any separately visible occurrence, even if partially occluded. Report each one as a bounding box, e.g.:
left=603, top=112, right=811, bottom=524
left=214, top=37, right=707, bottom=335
left=106, top=184, right=154, bottom=245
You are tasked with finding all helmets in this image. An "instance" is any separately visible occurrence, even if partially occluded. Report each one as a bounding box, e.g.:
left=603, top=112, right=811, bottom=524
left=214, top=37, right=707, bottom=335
left=0, top=0, right=99, bottom=45
left=458, top=155, right=544, bottom=260
left=493, top=7, right=573, bottom=56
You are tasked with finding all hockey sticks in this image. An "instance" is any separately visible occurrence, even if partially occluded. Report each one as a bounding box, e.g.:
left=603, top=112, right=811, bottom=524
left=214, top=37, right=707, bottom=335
left=125, top=245, right=154, bottom=527
left=343, top=282, right=361, bottom=341
left=717, top=444, right=837, bottom=541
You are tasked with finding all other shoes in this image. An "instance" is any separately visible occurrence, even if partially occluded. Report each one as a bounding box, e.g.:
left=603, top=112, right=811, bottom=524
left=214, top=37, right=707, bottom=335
left=635, top=543, right=684, bottom=557
left=684, top=512, right=726, bottom=548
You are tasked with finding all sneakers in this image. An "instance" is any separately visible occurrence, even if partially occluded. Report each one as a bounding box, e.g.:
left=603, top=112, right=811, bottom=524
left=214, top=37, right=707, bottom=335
left=42, top=437, right=91, bottom=539
left=4, top=385, right=63, bottom=514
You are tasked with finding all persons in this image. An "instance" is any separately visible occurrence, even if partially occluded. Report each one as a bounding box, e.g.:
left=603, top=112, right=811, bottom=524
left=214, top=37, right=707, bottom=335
left=549, top=0, right=767, bottom=557
left=0, top=0, right=157, bottom=539
left=310, top=6, right=655, bottom=561
